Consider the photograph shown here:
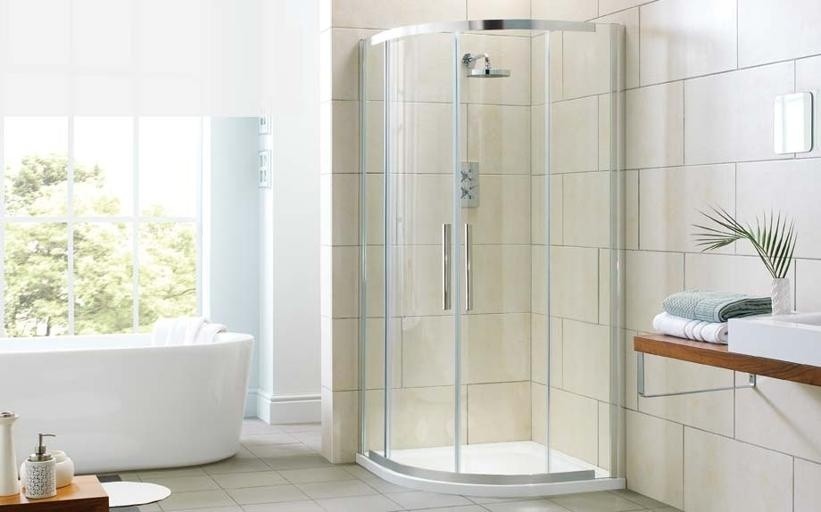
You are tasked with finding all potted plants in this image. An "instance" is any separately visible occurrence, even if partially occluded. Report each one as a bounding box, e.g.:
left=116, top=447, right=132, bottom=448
left=690, top=200, right=799, bottom=314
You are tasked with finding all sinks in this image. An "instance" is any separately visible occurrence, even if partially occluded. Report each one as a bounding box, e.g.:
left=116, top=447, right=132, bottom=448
left=728, top=309, right=821, bottom=366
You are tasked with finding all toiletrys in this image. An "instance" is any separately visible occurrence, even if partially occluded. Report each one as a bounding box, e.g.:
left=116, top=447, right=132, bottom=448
left=25, top=434, right=56, bottom=499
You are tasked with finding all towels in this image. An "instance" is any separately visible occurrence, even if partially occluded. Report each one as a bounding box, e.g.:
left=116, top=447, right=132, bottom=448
left=653, top=289, right=771, bottom=345
left=148, top=317, right=227, bottom=344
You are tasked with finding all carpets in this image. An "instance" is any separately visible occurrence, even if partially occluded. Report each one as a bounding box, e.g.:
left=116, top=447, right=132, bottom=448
left=97, top=475, right=172, bottom=512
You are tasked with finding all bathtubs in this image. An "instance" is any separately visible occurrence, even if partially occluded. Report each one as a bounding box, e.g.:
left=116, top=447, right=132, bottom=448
left=0, top=333, right=253, bottom=475
